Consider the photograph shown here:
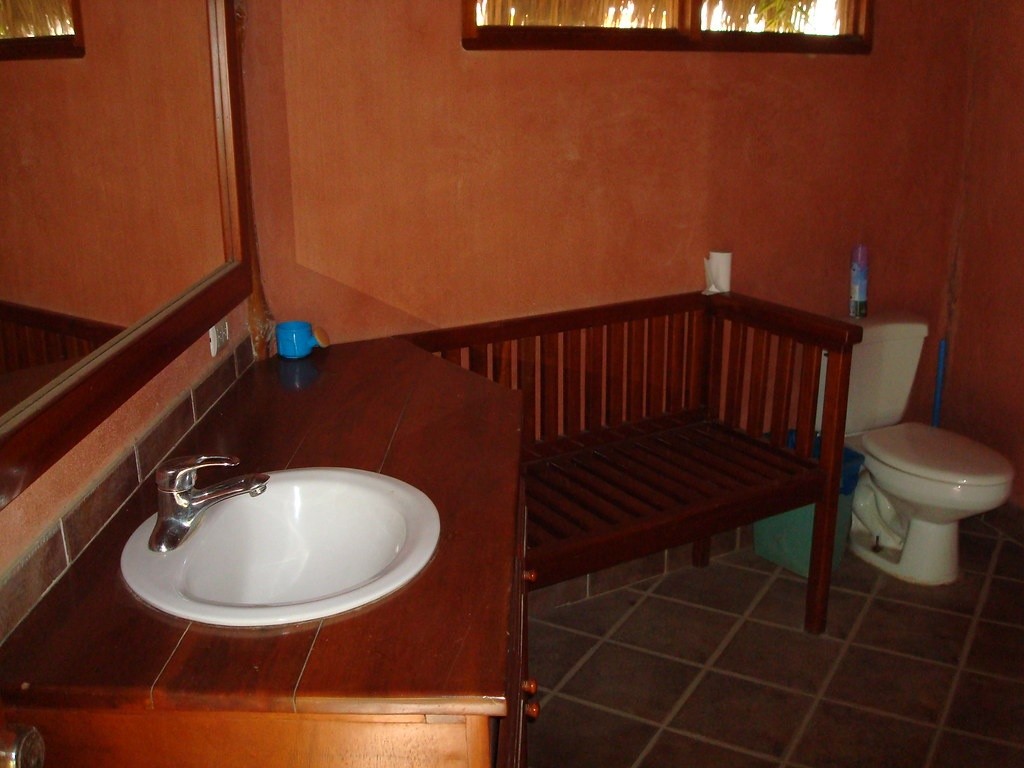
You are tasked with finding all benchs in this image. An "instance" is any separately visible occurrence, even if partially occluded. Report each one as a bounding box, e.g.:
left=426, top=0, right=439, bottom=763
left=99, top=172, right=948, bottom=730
left=396, top=288, right=866, bottom=637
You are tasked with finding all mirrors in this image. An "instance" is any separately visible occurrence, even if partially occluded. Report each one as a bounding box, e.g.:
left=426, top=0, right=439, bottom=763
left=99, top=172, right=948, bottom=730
left=1, top=1, right=236, bottom=427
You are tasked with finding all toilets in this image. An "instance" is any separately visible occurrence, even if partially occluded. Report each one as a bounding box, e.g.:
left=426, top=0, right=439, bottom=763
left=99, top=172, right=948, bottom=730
left=792, top=309, right=1017, bottom=589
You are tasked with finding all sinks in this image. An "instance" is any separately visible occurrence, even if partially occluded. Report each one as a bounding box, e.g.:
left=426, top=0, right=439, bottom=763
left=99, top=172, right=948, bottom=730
left=119, top=464, right=441, bottom=630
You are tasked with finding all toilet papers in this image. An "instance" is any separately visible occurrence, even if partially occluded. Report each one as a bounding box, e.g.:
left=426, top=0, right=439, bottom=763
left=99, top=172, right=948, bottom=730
left=703, top=249, right=735, bottom=298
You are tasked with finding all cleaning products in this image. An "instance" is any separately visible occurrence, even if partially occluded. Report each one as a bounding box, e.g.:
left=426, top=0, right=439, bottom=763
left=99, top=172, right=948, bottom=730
left=845, top=243, right=872, bottom=321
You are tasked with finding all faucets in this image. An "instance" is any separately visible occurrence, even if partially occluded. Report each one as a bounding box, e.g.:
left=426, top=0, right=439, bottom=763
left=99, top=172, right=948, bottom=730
left=144, top=457, right=273, bottom=552
